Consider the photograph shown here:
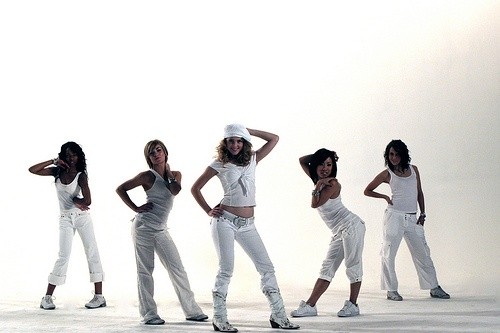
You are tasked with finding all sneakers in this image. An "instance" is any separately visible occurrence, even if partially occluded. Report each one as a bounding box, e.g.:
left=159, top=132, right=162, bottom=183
left=337, top=300, right=360, bottom=317
left=430, top=286, right=450, bottom=299
left=40, top=297, right=55, bottom=310
left=145, top=317, right=165, bottom=324
left=387, top=291, right=402, bottom=301
left=290, top=300, right=317, bottom=317
left=186, top=314, right=208, bottom=320
left=85, top=295, right=107, bottom=308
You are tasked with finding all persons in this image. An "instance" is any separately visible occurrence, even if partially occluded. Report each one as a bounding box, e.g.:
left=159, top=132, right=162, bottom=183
left=364, top=140, right=450, bottom=301
left=290, top=148, right=366, bottom=317
left=191, top=123, right=300, bottom=333
left=29, top=141, right=106, bottom=310
left=116, top=139, right=208, bottom=325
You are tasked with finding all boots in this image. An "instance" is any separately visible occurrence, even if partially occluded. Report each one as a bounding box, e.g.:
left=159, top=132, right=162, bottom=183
left=211, top=292, right=238, bottom=332
left=263, top=288, right=300, bottom=329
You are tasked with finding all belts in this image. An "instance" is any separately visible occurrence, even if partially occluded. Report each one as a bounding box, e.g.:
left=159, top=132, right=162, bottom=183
left=224, top=212, right=255, bottom=227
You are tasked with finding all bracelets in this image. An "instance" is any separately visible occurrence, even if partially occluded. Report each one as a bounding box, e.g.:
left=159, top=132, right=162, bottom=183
left=311, top=190, right=321, bottom=196
left=168, top=177, right=178, bottom=184
left=420, top=213, right=426, bottom=218
left=52, top=158, right=58, bottom=165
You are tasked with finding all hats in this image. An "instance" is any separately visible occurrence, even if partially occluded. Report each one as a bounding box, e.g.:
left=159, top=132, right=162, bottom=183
left=222, top=124, right=253, bottom=147
